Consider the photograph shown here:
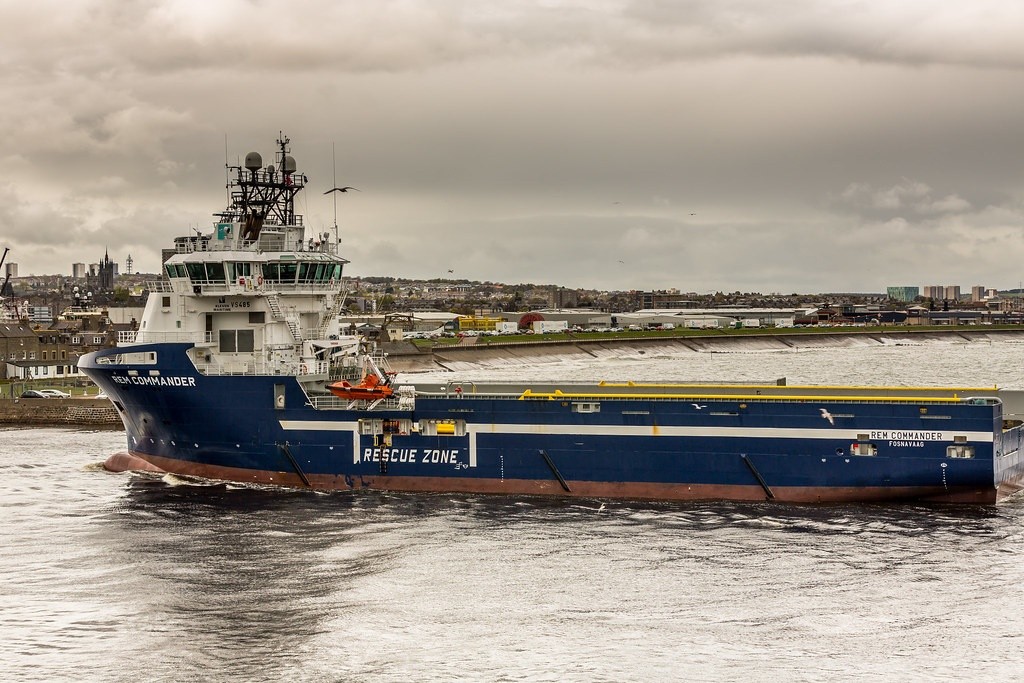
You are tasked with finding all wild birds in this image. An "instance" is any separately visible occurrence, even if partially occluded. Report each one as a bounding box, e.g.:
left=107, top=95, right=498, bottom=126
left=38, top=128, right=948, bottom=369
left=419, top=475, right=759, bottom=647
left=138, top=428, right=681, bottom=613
left=819, top=408, right=835, bottom=426
left=448, top=269, right=455, bottom=274
left=612, top=200, right=697, bottom=265
left=323, top=186, right=362, bottom=195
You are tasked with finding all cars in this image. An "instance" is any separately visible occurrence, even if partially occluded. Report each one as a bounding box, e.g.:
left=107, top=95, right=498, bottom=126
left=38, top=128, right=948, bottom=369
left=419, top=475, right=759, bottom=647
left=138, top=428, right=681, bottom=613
left=405, top=330, right=535, bottom=340
left=969, top=322, right=975, bottom=326
left=775, top=321, right=884, bottom=328
left=94, top=389, right=108, bottom=400
left=572, top=323, right=676, bottom=333
left=20, top=390, right=51, bottom=399
left=958, top=322, right=964, bottom=325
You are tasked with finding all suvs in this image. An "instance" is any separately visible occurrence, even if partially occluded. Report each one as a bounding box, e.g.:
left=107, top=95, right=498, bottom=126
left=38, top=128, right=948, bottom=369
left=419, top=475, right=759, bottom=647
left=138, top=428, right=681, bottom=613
left=39, top=389, right=72, bottom=399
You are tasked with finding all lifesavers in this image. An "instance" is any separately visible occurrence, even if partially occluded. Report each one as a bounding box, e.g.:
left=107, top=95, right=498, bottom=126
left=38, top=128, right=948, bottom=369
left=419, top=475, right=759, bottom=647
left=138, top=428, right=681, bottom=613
left=258, top=275, right=263, bottom=285
left=303, top=366, right=308, bottom=374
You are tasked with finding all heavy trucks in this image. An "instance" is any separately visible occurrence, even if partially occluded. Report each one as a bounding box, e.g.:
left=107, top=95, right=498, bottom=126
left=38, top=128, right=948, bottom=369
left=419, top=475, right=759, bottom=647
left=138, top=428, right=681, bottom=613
left=729, top=318, right=760, bottom=328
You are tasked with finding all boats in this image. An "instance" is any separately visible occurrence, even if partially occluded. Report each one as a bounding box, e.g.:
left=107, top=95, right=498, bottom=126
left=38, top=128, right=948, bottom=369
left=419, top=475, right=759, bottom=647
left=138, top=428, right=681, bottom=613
left=323, top=370, right=397, bottom=400
left=74, top=128, right=1024, bottom=511
